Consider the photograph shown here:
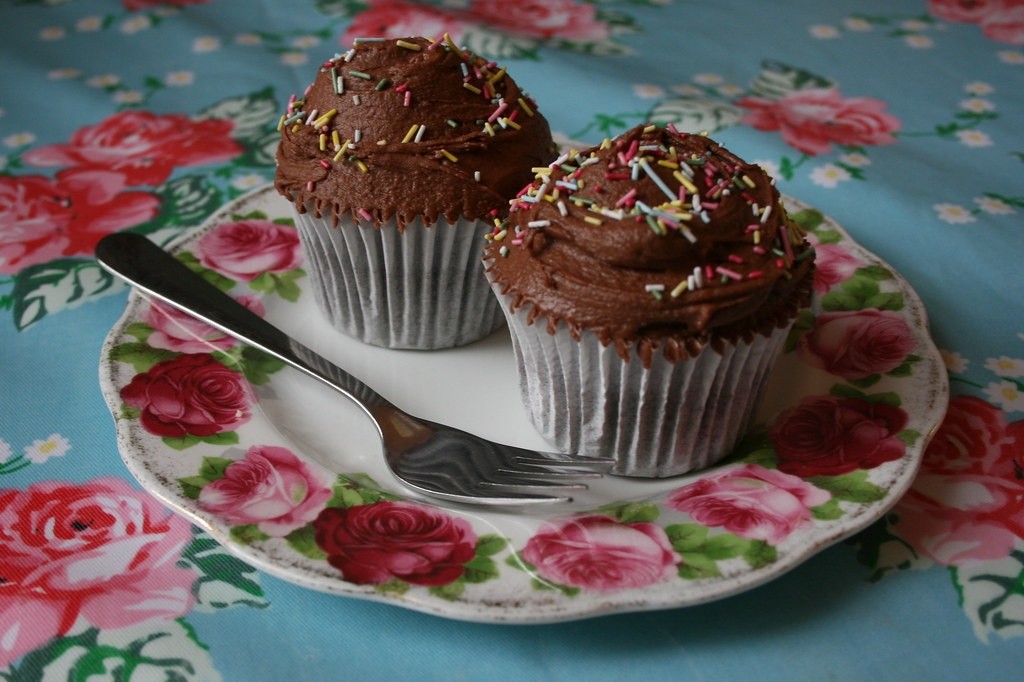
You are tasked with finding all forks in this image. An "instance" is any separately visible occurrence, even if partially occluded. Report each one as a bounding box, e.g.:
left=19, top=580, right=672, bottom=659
left=92, top=232, right=619, bottom=505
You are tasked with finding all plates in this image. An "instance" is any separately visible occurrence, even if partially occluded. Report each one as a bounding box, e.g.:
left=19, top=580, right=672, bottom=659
left=99, top=139, right=950, bottom=624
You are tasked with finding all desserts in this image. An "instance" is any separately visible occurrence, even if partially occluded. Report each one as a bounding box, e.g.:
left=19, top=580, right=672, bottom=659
left=481, top=121, right=816, bottom=479
left=275, top=32, right=561, bottom=350
